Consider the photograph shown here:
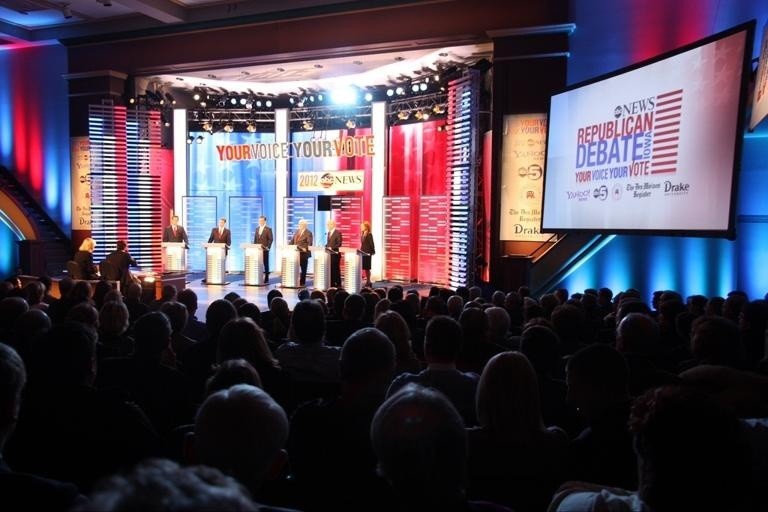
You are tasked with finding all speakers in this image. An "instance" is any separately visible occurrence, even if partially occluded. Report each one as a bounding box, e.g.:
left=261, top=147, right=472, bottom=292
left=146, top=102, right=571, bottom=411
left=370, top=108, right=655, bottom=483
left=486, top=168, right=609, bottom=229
left=19, top=240, right=45, bottom=277
left=494, top=257, right=531, bottom=296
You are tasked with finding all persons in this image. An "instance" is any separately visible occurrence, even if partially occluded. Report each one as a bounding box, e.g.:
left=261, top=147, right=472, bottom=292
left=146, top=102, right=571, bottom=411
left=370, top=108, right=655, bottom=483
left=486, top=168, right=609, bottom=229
left=254, top=216, right=273, bottom=283
left=106, top=241, right=138, bottom=280
left=162, top=216, right=188, bottom=250
left=73, top=237, right=100, bottom=279
left=2, top=275, right=768, bottom=511
left=207, top=218, right=232, bottom=255
left=289, top=219, right=313, bottom=286
left=325, top=219, right=342, bottom=287
left=359, top=221, right=375, bottom=288
